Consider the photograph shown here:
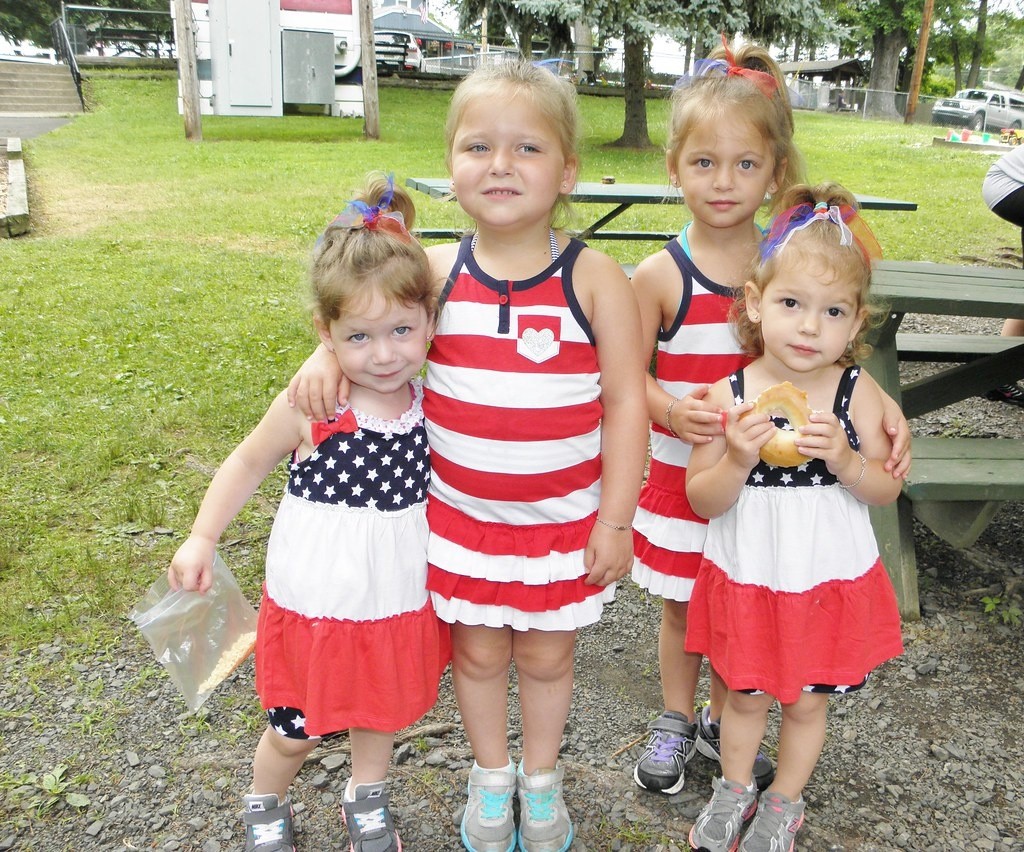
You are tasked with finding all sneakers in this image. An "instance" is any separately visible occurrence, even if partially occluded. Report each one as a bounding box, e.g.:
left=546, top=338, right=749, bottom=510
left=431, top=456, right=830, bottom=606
left=244, top=790, right=296, bottom=852
left=737, top=784, right=807, bottom=852
left=634, top=711, right=699, bottom=795
left=516, top=757, right=573, bottom=852
left=460, top=756, right=516, bottom=852
left=689, top=773, right=758, bottom=852
left=986, top=384, right=1024, bottom=407
left=342, top=776, right=402, bottom=852
left=696, top=706, right=774, bottom=786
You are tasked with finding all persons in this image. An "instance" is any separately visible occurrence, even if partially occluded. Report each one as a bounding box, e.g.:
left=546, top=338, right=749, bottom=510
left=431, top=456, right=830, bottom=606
left=631, top=31, right=911, bottom=795
left=286, top=60, right=647, bottom=852
left=685, top=183, right=904, bottom=852
left=982, top=142, right=1024, bottom=406
left=167, top=174, right=440, bottom=852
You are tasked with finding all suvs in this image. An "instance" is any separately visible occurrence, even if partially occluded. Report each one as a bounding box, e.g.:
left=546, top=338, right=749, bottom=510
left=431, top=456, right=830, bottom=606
left=929, top=87, right=1024, bottom=135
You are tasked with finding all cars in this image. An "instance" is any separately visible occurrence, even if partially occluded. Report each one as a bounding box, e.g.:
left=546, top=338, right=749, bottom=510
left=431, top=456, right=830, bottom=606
left=374, top=29, right=428, bottom=73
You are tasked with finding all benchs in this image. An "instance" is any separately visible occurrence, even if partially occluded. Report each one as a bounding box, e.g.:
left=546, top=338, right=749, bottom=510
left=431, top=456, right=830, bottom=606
left=165, top=27, right=176, bottom=59
left=94, top=22, right=162, bottom=58
left=868, top=437, right=1024, bottom=621
left=845, top=331, right=1024, bottom=363
left=404, top=229, right=679, bottom=239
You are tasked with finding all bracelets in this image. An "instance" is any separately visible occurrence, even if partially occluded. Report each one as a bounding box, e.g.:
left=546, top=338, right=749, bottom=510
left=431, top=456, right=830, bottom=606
left=666, top=396, right=682, bottom=439
left=836, top=450, right=866, bottom=489
left=596, top=515, right=634, bottom=532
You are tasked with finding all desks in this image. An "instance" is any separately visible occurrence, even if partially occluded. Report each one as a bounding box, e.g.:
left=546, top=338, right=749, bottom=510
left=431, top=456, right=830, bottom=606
left=404, top=177, right=919, bottom=241
left=847, top=258, right=1024, bottom=416
